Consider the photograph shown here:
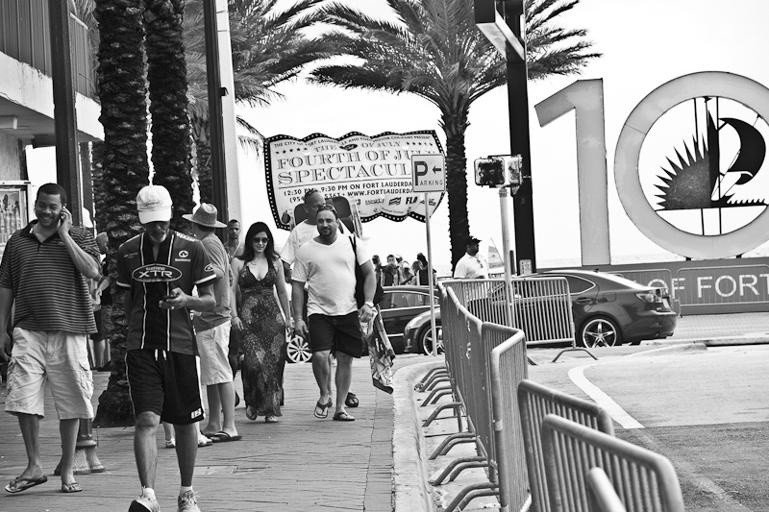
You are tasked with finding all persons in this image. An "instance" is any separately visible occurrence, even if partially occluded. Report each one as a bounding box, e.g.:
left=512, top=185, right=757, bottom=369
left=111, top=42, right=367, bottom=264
left=180, top=203, right=245, bottom=442
left=291, top=205, right=377, bottom=421
left=372, top=253, right=434, bottom=305
left=83, top=207, right=111, bottom=370
left=281, top=189, right=361, bottom=409
left=0, top=185, right=98, bottom=493
left=117, top=186, right=217, bottom=512
left=222, top=220, right=245, bottom=263
left=453, top=237, right=490, bottom=321
left=163, top=421, right=213, bottom=447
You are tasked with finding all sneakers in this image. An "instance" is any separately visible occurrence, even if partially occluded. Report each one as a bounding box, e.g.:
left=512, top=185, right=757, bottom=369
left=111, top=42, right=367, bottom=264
left=178, top=490, right=200, bottom=512
left=128, top=496, right=160, bottom=512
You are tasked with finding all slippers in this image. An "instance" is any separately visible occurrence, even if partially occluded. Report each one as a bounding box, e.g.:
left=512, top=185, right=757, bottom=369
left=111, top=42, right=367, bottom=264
left=62, top=482, right=82, bottom=493
left=246, top=405, right=278, bottom=423
left=165, top=430, right=241, bottom=448
left=314, top=392, right=358, bottom=421
left=5, top=476, right=47, bottom=493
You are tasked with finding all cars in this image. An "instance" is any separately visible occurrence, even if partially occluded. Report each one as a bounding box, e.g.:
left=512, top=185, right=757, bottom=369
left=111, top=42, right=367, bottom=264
left=281, top=285, right=444, bottom=362
left=403, top=270, right=677, bottom=353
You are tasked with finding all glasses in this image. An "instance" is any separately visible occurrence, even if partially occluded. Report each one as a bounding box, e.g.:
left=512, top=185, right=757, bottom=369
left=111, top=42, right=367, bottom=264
left=254, top=237, right=269, bottom=243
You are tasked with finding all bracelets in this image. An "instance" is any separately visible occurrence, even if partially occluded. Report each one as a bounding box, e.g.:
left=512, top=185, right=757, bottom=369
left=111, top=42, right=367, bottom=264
left=364, top=300, right=374, bottom=307
left=232, top=315, right=239, bottom=320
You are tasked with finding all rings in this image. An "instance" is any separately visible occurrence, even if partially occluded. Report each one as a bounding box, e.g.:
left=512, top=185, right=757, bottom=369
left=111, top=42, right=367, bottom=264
left=171, top=306, right=174, bottom=310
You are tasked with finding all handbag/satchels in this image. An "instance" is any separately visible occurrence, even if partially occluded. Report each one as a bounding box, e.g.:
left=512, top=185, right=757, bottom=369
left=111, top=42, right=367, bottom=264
left=355, top=261, right=383, bottom=309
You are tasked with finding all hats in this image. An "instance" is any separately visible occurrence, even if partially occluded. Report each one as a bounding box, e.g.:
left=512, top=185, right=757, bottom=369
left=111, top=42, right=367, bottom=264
left=465, top=236, right=480, bottom=244
left=182, top=203, right=228, bottom=228
left=136, top=185, right=173, bottom=224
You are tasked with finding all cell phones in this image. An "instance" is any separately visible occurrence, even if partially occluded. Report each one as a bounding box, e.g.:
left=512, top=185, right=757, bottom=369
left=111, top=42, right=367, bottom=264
left=60, top=205, right=66, bottom=219
left=162, top=295, right=177, bottom=303
